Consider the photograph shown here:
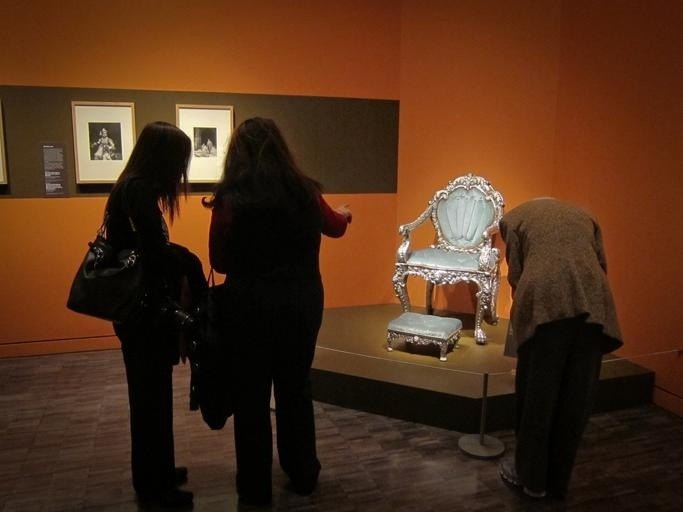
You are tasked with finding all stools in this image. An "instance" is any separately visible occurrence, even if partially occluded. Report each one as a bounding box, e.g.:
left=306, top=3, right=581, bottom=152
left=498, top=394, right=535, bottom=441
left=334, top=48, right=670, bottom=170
left=385, top=310, right=463, bottom=361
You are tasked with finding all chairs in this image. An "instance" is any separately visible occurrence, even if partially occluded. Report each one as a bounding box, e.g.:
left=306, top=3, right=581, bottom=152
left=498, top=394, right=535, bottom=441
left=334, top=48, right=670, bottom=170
left=391, top=171, right=506, bottom=345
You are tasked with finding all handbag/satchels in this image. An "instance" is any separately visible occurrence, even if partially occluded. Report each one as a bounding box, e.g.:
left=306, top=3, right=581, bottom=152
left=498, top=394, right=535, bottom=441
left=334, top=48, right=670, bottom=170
left=66, top=217, right=147, bottom=330
left=188, top=267, right=236, bottom=431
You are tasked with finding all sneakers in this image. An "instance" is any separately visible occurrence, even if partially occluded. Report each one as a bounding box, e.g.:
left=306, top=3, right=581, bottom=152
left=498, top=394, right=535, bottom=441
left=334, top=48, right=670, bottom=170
left=500, top=464, right=545, bottom=499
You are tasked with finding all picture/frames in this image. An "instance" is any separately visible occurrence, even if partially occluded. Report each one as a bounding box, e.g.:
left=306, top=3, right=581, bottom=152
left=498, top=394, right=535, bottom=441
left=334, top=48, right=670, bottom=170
left=174, top=103, right=236, bottom=186
left=69, top=98, right=140, bottom=186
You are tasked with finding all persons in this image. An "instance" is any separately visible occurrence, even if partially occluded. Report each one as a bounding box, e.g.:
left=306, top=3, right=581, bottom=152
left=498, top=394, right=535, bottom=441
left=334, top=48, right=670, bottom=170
left=194, top=136, right=216, bottom=157
left=103, top=122, right=201, bottom=511
left=498, top=197, right=624, bottom=510
left=202, top=116, right=353, bottom=511
left=92, top=127, right=117, bottom=160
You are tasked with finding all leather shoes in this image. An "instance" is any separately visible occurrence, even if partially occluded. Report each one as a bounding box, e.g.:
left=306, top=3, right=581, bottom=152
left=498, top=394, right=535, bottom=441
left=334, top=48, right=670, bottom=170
left=181, top=491, right=194, bottom=507
left=174, top=465, right=187, bottom=485
